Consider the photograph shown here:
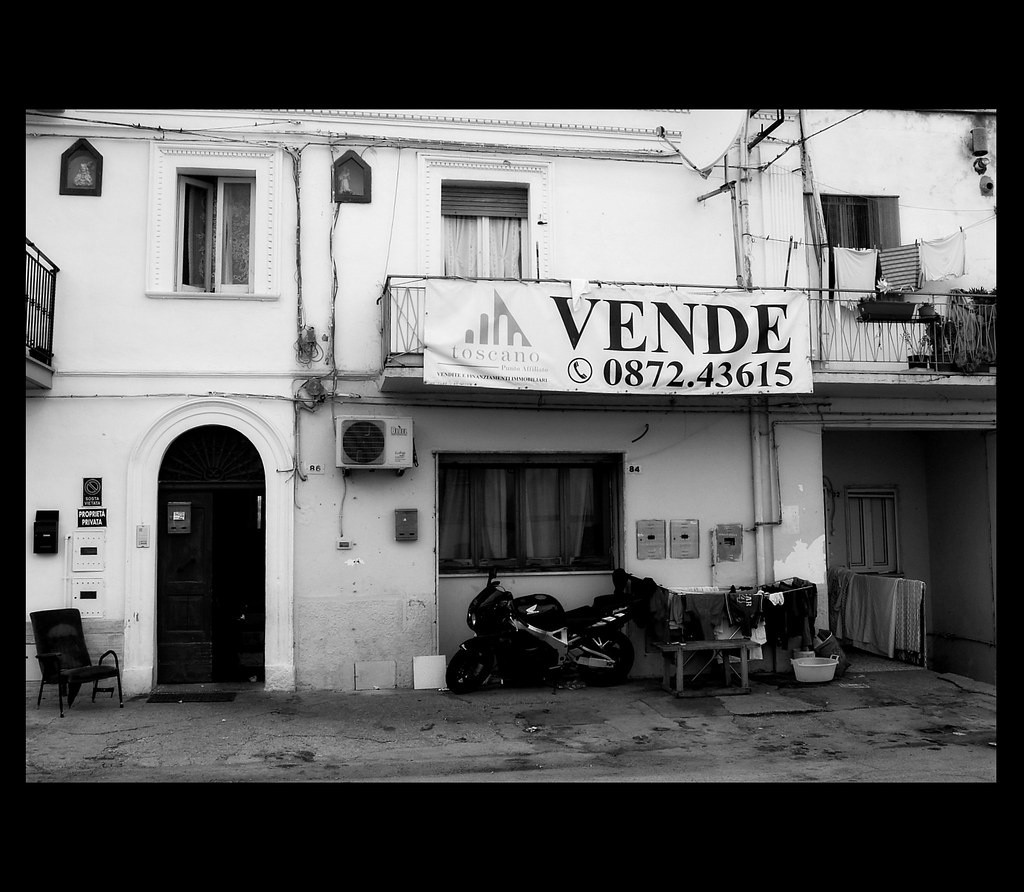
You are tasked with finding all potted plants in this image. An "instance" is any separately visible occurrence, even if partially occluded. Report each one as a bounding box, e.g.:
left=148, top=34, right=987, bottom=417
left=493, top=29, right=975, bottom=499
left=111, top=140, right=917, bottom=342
left=899, top=331, right=931, bottom=369
left=857, top=292, right=916, bottom=320
left=918, top=298, right=935, bottom=319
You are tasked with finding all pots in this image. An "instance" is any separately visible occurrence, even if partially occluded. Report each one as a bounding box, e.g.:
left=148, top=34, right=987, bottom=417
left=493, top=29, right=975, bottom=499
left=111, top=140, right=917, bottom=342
left=790, top=655, right=840, bottom=683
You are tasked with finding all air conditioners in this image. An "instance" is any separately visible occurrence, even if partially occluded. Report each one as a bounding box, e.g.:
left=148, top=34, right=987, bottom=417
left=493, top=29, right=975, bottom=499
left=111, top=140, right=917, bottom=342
left=335, top=415, right=414, bottom=469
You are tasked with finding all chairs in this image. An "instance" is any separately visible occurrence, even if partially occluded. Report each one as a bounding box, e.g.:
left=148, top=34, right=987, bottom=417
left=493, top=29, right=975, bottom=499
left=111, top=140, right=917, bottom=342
left=29, top=608, right=125, bottom=718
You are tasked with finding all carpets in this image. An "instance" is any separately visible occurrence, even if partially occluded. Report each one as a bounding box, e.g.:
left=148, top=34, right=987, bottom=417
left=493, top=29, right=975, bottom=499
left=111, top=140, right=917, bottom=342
left=146, top=691, right=238, bottom=703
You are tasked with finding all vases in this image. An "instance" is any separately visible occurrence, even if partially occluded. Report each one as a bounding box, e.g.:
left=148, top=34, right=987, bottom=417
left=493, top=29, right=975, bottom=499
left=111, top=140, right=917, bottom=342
left=881, top=291, right=904, bottom=302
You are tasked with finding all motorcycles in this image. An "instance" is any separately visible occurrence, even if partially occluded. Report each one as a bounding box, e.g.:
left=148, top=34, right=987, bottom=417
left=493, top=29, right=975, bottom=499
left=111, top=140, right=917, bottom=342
left=445, top=567, right=638, bottom=695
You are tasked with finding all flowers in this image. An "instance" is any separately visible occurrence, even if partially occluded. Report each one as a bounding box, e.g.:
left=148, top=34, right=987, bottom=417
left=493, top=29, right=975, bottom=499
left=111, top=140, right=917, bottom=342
left=876, top=278, right=894, bottom=295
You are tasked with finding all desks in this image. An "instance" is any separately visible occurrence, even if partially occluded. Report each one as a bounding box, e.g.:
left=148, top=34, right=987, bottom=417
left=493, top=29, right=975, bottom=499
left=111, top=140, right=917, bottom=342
left=652, top=638, right=760, bottom=699
